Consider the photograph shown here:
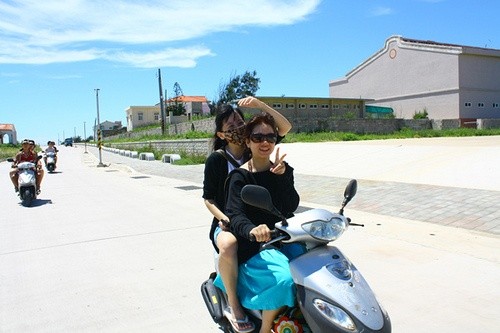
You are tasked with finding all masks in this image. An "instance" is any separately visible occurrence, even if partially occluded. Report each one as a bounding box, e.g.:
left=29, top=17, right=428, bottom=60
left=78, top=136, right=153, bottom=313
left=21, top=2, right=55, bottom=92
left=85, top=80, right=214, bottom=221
left=222, top=124, right=248, bottom=146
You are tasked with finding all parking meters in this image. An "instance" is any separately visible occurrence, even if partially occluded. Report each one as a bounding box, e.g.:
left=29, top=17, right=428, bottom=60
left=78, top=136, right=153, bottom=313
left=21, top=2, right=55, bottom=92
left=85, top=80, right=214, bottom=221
left=94, top=88, right=103, bottom=167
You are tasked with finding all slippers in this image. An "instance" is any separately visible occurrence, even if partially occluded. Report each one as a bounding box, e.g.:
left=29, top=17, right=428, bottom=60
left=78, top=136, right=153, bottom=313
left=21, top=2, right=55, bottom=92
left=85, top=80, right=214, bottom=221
left=223, top=307, right=256, bottom=333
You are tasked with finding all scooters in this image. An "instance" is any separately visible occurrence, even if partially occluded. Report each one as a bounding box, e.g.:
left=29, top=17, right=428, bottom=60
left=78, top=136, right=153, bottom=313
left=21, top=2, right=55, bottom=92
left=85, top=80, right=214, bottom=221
left=200, top=179, right=392, bottom=333
left=42, top=150, right=59, bottom=173
left=6, top=155, right=43, bottom=206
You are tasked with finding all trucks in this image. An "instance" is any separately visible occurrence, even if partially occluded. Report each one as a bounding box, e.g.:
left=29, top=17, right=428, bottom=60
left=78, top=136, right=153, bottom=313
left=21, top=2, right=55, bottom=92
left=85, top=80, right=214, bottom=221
left=65, top=138, right=72, bottom=147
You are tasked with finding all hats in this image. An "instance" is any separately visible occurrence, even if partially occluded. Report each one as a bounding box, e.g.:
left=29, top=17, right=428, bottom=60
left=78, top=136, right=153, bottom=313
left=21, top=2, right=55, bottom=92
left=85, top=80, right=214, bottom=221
left=29, top=140, right=35, bottom=145
left=22, top=139, right=29, bottom=144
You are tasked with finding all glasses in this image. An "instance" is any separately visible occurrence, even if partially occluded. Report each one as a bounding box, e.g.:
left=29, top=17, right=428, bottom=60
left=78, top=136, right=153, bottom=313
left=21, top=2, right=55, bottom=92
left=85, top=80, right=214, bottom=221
left=249, top=132, right=277, bottom=143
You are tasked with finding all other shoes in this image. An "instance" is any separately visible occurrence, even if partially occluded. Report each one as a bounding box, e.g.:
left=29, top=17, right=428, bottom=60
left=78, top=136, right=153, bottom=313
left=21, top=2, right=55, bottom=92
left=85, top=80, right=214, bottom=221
left=36, top=189, right=40, bottom=195
left=15, top=187, right=19, bottom=192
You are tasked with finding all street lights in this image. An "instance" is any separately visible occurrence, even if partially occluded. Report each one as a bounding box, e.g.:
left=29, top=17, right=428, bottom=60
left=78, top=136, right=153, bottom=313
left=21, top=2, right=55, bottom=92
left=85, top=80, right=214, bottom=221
left=82, top=121, right=89, bottom=153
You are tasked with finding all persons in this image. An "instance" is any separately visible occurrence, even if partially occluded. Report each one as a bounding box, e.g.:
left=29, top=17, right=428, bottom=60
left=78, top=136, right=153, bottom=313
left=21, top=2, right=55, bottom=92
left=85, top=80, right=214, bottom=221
left=52, top=142, right=56, bottom=153
left=213, top=111, right=299, bottom=333
left=202, top=95, right=292, bottom=333
left=20, top=140, right=42, bottom=169
left=9, top=139, right=44, bottom=197
left=43, top=141, right=57, bottom=168
